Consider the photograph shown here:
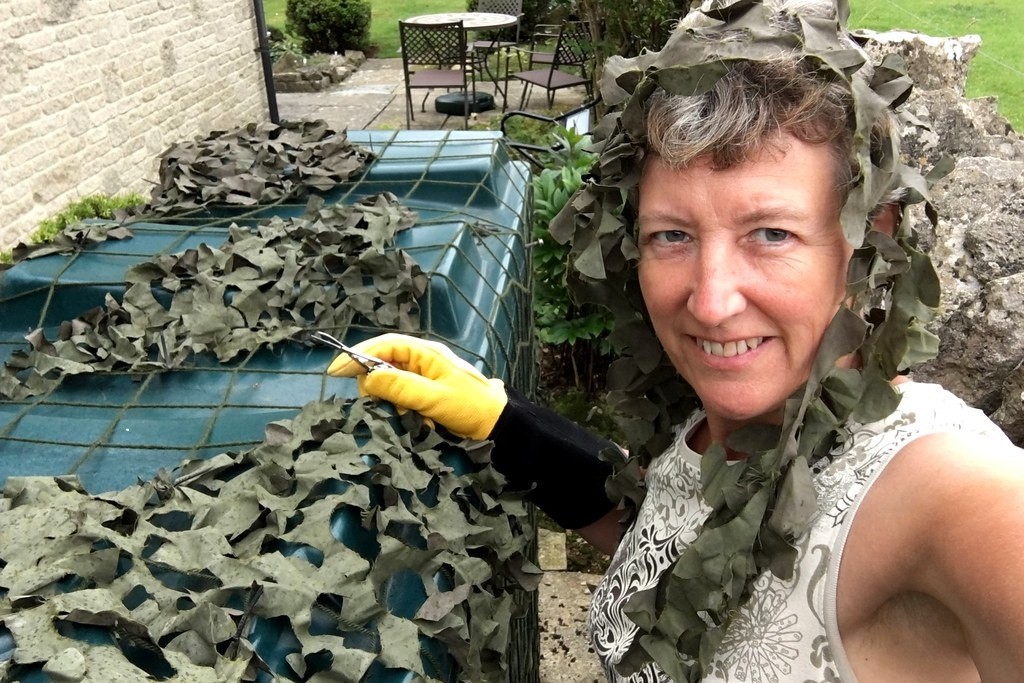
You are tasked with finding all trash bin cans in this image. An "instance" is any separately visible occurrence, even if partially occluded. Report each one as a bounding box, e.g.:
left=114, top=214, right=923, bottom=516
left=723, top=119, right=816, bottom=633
left=0, top=127, right=535, bottom=683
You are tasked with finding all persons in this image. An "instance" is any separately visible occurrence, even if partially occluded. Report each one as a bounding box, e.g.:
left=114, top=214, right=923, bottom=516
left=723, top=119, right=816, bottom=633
left=327, top=0, right=1024, bottom=683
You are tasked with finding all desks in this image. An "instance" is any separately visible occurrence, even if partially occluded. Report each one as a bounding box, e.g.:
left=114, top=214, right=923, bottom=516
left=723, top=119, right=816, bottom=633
left=405, top=12, right=518, bottom=114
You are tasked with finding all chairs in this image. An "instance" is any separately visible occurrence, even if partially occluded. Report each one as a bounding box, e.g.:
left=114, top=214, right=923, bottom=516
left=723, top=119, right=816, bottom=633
left=447, top=0, right=524, bottom=96
left=525, top=24, right=590, bottom=109
left=397, top=18, right=478, bottom=131
left=508, top=18, right=605, bottom=111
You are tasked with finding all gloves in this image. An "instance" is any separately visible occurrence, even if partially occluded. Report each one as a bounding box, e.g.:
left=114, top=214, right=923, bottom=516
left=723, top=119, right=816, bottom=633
left=327, top=333, right=508, bottom=444
